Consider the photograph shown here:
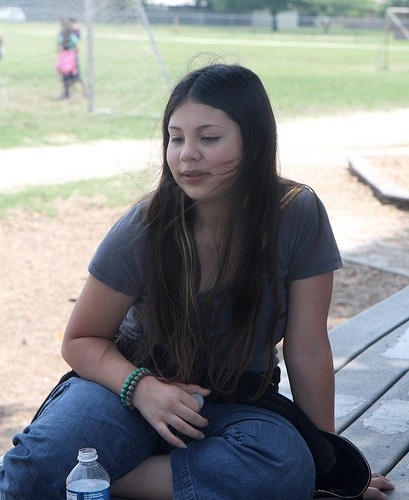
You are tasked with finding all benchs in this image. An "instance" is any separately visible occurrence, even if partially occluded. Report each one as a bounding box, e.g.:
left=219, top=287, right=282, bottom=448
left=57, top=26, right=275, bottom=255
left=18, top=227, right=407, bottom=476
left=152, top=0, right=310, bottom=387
left=278, top=285, right=409, bottom=500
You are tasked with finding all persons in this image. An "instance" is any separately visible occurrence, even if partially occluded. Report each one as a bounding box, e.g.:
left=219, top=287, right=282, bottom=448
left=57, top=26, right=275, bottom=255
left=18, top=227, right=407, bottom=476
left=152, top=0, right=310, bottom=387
left=56, top=39, right=88, bottom=99
left=61, top=18, right=81, bottom=49
left=0, top=65, right=395, bottom=500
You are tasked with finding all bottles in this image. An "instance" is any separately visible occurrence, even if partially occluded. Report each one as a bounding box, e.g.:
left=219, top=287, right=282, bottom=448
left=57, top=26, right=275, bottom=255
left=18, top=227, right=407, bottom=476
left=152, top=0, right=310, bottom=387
left=66, top=448, right=111, bottom=500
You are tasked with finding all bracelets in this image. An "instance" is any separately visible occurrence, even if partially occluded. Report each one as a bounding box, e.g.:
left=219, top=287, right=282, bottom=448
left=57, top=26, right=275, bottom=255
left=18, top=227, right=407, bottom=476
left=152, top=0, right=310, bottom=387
left=120, top=367, right=147, bottom=407
left=125, top=370, right=154, bottom=409
